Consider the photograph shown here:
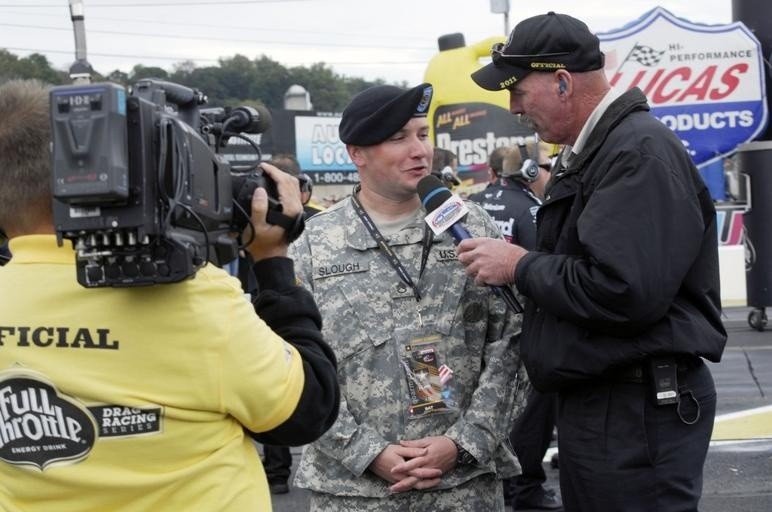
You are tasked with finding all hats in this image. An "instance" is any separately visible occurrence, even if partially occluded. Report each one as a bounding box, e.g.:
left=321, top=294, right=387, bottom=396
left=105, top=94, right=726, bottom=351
left=470, top=11, right=607, bottom=91
left=338, top=83, right=433, bottom=148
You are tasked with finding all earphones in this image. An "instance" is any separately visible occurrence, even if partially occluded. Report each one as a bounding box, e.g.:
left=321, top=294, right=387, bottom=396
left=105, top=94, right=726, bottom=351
left=558, top=80, right=568, bottom=95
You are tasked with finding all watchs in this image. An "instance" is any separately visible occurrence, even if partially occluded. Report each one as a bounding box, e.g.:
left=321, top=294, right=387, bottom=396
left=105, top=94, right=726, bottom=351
left=451, top=439, right=475, bottom=467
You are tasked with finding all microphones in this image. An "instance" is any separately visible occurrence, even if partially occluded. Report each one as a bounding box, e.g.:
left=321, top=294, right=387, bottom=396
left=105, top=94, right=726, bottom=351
left=415, top=175, right=523, bottom=317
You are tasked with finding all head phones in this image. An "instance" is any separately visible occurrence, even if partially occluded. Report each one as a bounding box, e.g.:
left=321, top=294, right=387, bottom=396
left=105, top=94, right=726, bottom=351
left=439, top=166, right=460, bottom=186
left=510, top=142, right=540, bottom=181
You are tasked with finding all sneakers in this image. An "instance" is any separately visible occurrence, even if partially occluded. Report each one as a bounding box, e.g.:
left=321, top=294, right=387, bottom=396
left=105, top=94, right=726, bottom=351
left=512, top=486, right=563, bottom=511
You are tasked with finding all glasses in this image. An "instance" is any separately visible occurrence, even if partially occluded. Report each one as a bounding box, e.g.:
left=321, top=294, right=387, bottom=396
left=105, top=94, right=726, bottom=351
left=490, top=41, right=570, bottom=68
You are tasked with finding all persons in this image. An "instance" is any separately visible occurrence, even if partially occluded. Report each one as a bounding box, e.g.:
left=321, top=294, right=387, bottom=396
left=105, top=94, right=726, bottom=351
left=2, top=79, right=340, bottom=512
left=238, top=155, right=324, bottom=495
left=432, top=149, right=460, bottom=191
left=286, top=85, right=532, bottom=510
left=454, top=12, right=727, bottom=510
left=487, top=144, right=509, bottom=182
left=468, top=141, right=551, bottom=252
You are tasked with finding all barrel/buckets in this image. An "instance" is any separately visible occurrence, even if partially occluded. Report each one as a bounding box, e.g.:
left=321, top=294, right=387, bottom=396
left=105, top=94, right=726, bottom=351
left=424, top=33, right=559, bottom=194
left=736, top=140, right=772, bottom=331
